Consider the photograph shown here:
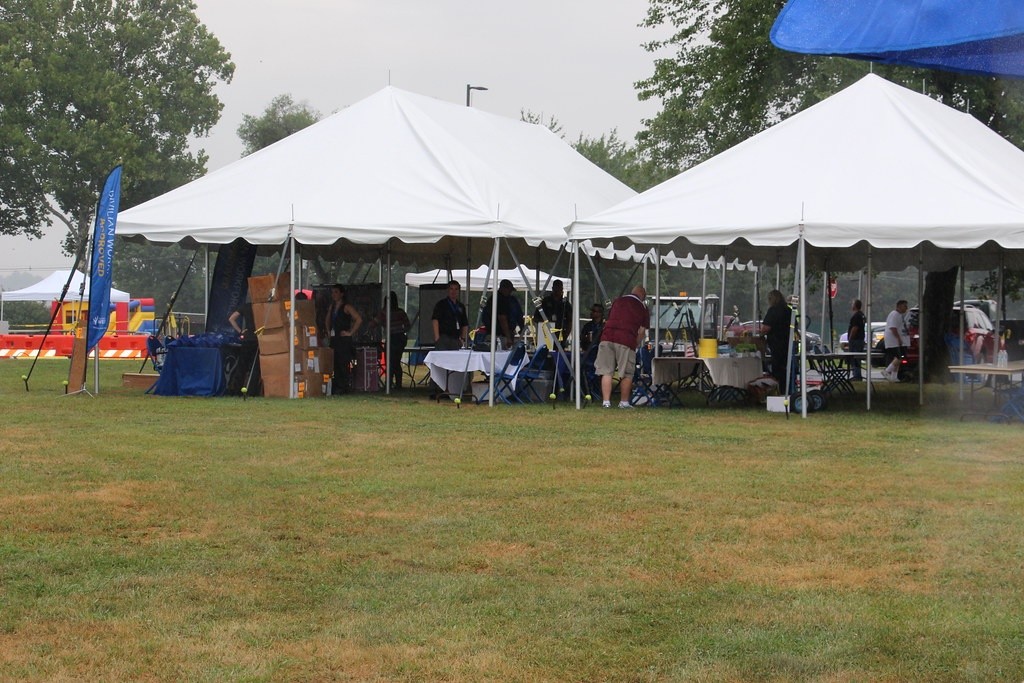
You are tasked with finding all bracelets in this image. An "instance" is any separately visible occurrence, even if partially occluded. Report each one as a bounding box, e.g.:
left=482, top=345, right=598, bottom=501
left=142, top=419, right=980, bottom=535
left=240, top=329, right=242, bottom=334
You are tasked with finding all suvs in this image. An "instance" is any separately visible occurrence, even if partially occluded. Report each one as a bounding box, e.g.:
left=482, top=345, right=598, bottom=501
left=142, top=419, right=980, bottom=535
left=897, top=304, right=1005, bottom=381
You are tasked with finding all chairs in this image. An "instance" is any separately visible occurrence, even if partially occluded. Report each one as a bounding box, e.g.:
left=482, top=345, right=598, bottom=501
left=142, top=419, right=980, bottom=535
left=994, top=386, right=1024, bottom=423
left=147, top=336, right=164, bottom=375
left=476, top=341, right=526, bottom=406
left=514, top=343, right=547, bottom=404
left=554, top=343, right=663, bottom=406
left=811, top=343, right=856, bottom=397
left=164, top=334, right=177, bottom=348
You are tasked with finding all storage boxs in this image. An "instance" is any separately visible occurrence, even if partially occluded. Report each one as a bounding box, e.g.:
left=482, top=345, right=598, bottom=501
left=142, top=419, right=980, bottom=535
left=248, top=272, right=334, bottom=397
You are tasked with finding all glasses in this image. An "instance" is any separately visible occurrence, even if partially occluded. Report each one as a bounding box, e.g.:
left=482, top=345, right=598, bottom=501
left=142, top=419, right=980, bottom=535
left=901, top=305, right=907, bottom=309
left=590, top=309, right=600, bottom=313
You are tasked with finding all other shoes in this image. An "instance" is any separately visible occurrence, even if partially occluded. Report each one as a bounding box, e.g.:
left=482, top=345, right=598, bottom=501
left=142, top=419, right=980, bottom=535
left=429, top=394, right=436, bottom=400
left=850, top=373, right=862, bottom=381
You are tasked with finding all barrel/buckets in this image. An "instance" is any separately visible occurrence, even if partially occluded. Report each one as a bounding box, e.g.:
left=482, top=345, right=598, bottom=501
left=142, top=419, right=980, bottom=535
left=698, top=338, right=717, bottom=359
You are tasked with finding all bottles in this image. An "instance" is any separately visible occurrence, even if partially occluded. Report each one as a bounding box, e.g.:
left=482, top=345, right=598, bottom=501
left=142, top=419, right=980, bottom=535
left=497, top=341, right=502, bottom=350
left=997, top=350, right=1007, bottom=367
left=798, top=340, right=801, bottom=352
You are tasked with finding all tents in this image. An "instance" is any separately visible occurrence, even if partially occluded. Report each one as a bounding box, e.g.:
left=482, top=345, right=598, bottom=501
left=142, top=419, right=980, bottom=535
left=562, top=73, right=1024, bottom=418
left=1, top=269, right=130, bottom=336
left=88, top=85, right=758, bottom=407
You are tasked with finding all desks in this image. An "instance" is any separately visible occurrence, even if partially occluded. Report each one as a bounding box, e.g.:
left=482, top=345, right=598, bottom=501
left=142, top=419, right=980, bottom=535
left=948, top=360, right=1024, bottom=422
left=799, top=352, right=885, bottom=399
left=651, top=353, right=763, bottom=407
left=424, top=348, right=530, bottom=404
left=400, top=346, right=436, bottom=391
left=145, top=346, right=223, bottom=396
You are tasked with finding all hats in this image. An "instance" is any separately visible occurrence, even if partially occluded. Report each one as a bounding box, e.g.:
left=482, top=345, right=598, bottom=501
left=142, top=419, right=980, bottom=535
left=500, top=280, right=517, bottom=291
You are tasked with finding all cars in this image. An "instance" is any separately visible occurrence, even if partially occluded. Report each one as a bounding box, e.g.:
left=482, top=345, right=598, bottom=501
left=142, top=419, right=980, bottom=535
left=839, top=322, right=886, bottom=352
left=954, top=300, right=998, bottom=321
left=734, top=321, right=821, bottom=354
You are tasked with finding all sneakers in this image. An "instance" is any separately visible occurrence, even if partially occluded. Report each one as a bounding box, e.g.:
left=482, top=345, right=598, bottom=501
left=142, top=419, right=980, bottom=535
left=618, top=401, right=633, bottom=409
left=602, top=401, right=612, bottom=408
left=880, top=370, right=900, bottom=383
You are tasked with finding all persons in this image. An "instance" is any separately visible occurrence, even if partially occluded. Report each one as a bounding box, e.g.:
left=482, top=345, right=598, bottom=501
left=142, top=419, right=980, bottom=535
left=296, top=284, right=363, bottom=393
left=429, top=280, right=468, bottom=400
left=847, top=299, right=867, bottom=381
left=580, top=285, right=650, bottom=409
left=761, top=289, right=798, bottom=411
left=480, top=279, right=572, bottom=351
left=228, top=291, right=260, bottom=397
left=881, top=300, right=908, bottom=383
left=357, top=291, right=411, bottom=389
